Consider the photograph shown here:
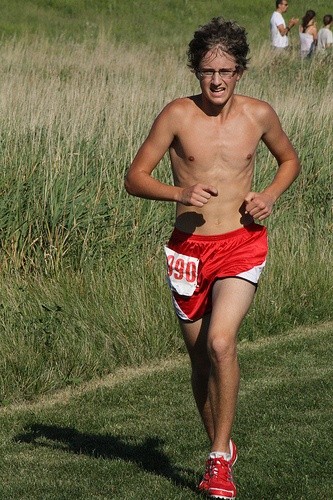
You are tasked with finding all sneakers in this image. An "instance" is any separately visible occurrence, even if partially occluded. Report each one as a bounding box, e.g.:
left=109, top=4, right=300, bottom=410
left=200, top=439, right=237, bottom=490
left=200, top=458, right=235, bottom=500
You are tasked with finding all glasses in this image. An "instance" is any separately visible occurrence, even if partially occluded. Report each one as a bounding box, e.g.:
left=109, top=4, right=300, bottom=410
left=281, top=4, right=288, bottom=6
left=194, top=65, right=237, bottom=79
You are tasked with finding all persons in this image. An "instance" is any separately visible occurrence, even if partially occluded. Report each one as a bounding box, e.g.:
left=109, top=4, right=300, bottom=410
left=124, top=17, right=301, bottom=500
left=298, top=9, right=317, bottom=62
left=317, top=15, right=333, bottom=56
left=270, top=0, right=299, bottom=63
